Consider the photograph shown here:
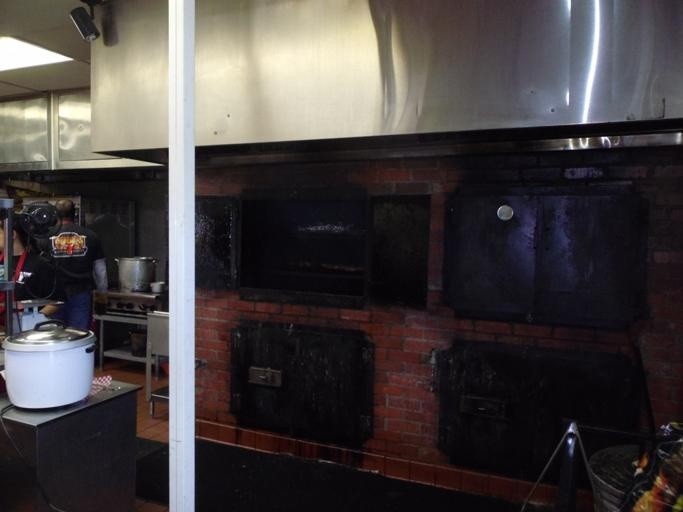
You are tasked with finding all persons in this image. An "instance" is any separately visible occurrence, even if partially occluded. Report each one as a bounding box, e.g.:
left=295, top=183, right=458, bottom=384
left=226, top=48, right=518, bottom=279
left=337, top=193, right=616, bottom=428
left=0, top=200, right=107, bottom=330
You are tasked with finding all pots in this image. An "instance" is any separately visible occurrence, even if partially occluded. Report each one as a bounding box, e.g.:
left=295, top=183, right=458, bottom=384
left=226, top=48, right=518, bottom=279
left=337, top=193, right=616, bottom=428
left=113, top=255, right=158, bottom=293
left=3, top=319, right=98, bottom=411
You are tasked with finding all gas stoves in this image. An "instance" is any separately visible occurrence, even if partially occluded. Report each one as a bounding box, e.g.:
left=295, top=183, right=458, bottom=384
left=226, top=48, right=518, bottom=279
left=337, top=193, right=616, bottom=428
left=93, top=288, right=168, bottom=319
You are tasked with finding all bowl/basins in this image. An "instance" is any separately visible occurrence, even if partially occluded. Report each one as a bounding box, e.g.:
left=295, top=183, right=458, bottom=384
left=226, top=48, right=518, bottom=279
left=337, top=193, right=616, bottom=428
left=151, top=282, right=165, bottom=293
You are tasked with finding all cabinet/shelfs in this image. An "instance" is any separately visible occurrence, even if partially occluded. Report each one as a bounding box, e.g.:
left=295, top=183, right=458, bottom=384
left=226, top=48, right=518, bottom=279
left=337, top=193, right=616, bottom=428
left=0, top=199, right=15, bottom=339
